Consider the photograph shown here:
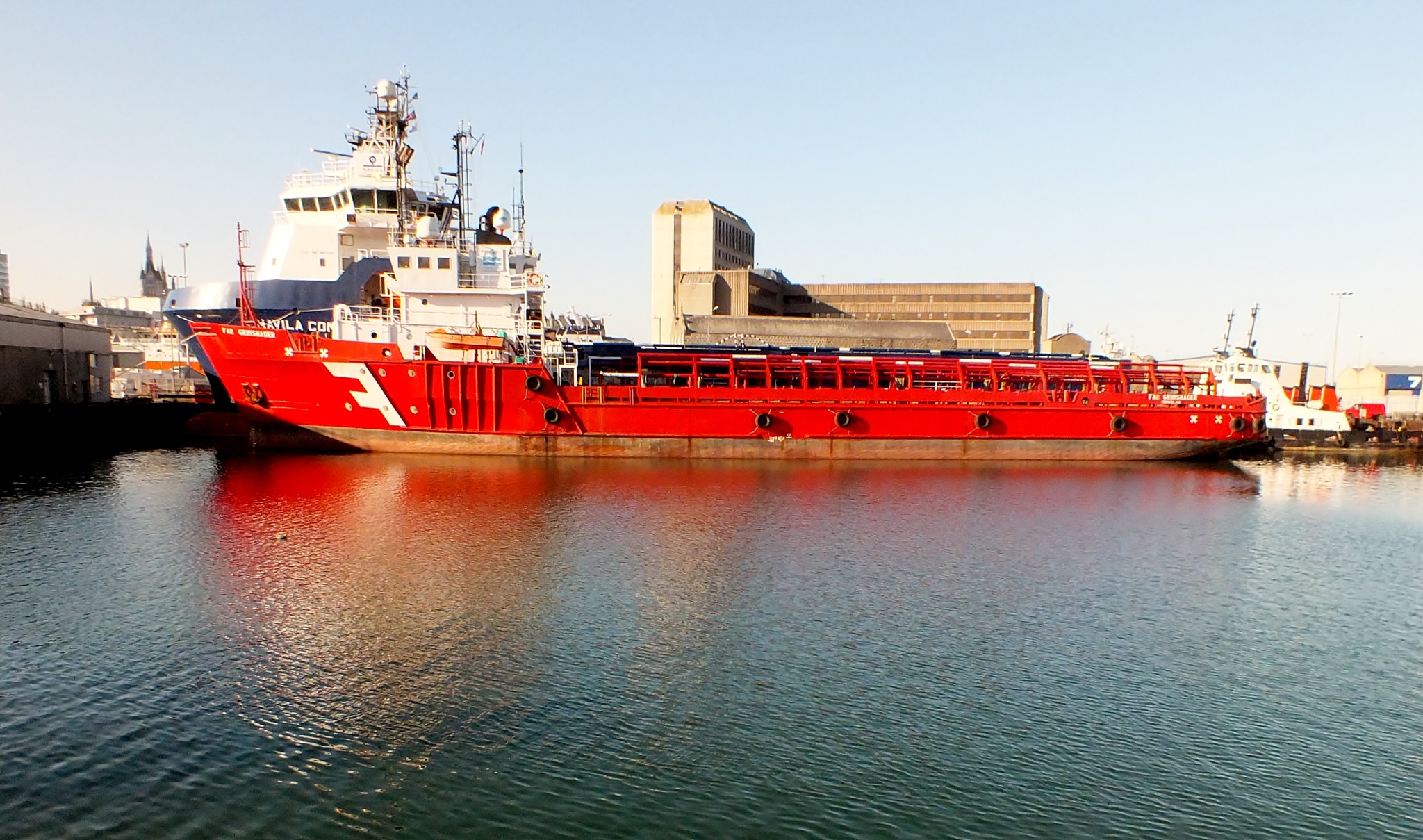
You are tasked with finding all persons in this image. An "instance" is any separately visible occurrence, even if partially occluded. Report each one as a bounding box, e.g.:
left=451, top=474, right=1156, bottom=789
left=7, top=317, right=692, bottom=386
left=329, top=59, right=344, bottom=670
left=1365, top=420, right=1407, bottom=443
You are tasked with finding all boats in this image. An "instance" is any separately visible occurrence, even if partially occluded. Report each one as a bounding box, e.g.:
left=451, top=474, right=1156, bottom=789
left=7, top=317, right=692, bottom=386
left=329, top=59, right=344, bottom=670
left=1192, top=306, right=1352, bottom=441
left=161, top=57, right=606, bottom=448
left=185, top=121, right=1273, bottom=461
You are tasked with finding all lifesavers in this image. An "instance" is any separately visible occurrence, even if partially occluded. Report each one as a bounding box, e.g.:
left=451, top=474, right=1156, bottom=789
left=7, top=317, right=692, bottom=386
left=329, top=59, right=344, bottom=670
left=1229, top=416, right=1246, bottom=432
left=525, top=374, right=542, bottom=392
left=755, top=412, right=771, bottom=428
left=835, top=411, right=851, bottom=427
left=544, top=407, right=560, bottom=424
left=1252, top=418, right=1266, bottom=433
left=1110, top=416, right=1128, bottom=432
left=974, top=413, right=990, bottom=429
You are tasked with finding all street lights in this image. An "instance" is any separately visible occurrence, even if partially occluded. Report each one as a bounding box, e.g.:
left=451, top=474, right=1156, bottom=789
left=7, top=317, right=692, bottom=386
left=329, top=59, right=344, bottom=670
left=177, top=275, right=188, bottom=377
left=567, top=311, right=612, bottom=342
left=178, top=242, right=190, bottom=288
left=1330, top=291, right=1354, bottom=384
left=653, top=317, right=661, bottom=345
left=168, top=275, right=175, bottom=388
